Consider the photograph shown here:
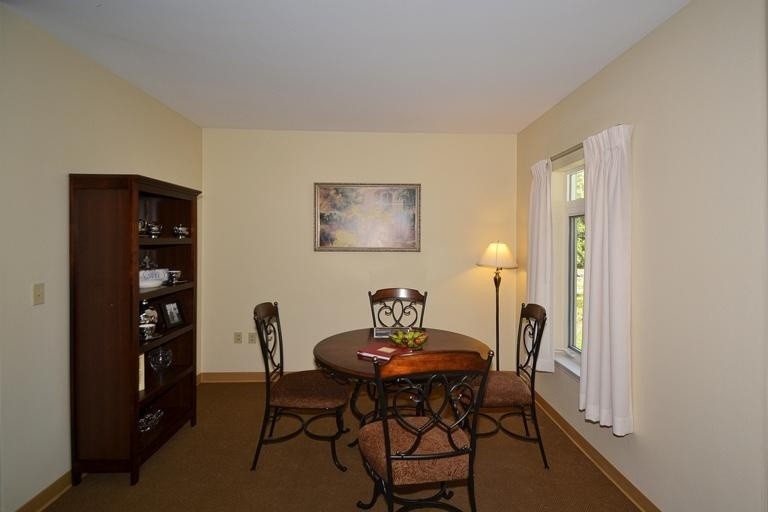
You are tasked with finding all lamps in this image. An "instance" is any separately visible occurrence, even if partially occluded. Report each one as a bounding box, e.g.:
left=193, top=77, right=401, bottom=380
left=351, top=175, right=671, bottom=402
left=473, top=240, right=520, bottom=370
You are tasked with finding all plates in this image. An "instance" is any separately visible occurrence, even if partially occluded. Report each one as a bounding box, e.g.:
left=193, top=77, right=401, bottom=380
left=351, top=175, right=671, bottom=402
left=168, top=280, right=188, bottom=283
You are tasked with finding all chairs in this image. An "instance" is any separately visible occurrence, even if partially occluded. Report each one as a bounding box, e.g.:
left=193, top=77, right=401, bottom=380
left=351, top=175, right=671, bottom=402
left=368, top=287, right=427, bottom=333
left=352, top=349, right=495, bottom=512
left=250, top=302, right=350, bottom=472
left=454, top=303, right=549, bottom=470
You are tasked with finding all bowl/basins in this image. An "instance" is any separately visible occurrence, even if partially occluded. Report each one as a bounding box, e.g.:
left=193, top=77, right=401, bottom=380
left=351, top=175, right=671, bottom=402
left=388, top=332, right=429, bottom=350
left=139, top=323, right=156, bottom=336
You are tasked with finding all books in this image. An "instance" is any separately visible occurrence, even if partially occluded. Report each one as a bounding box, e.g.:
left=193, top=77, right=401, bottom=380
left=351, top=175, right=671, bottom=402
left=356, top=339, right=413, bottom=362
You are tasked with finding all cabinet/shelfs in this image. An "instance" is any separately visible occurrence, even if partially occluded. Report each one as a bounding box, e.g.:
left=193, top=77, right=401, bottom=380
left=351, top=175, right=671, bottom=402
left=68, top=173, right=203, bottom=486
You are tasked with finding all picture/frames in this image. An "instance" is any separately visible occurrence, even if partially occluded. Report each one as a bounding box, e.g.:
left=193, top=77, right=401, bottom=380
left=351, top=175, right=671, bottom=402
left=312, top=182, right=421, bottom=253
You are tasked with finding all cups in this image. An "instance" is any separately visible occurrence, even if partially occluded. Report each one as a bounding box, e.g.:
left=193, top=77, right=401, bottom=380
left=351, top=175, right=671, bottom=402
left=168, top=270, right=181, bottom=278
left=150, top=223, right=163, bottom=234
left=139, top=218, right=148, bottom=232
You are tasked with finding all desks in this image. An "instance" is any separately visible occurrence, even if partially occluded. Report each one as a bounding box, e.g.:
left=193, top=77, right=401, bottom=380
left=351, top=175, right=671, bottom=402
left=313, top=328, right=491, bottom=449
